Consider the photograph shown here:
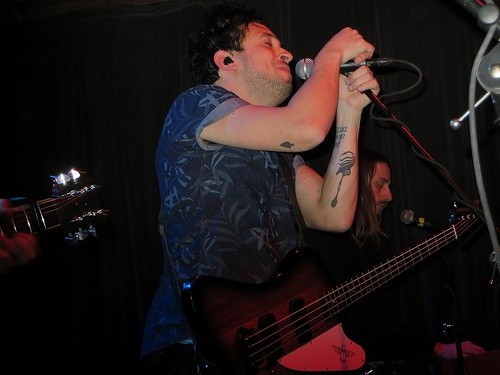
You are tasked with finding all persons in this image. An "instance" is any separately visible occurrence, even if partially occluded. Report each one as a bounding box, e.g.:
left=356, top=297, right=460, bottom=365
left=155, top=14, right=382, bottom=374
left=305, top=149, right=485, bottom=375
left=0, top=214, right=42, bottom=263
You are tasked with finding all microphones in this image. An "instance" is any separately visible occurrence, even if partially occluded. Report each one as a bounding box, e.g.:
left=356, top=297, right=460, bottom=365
left=296, top=57, right=394, bottom=80
left=400, top=210, right=443, bottom=233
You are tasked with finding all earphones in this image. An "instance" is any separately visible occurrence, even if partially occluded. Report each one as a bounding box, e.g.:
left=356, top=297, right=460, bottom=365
left=224, top=57, right=233, bottom=65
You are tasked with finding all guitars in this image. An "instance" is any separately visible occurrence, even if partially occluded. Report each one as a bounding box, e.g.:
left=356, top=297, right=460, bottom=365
left=1, top=166, right=111, bottom=256
left=152, top=198, right=478, bottom=374
